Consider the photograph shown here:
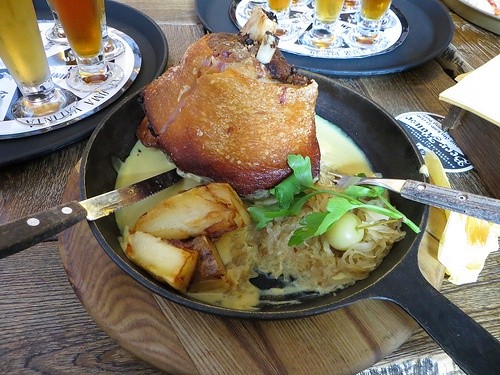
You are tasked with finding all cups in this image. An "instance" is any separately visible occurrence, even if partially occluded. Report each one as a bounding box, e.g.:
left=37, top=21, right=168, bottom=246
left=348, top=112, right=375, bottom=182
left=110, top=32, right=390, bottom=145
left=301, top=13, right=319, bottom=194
left=304, top=0, right=344, bottom=51
left=266, top=1, right=294, bottom=40
left=348, top=1, right=398, bottom=51
left=47, top=1, right=124, bottom=91
left=0, top=1, right=77, bottom=126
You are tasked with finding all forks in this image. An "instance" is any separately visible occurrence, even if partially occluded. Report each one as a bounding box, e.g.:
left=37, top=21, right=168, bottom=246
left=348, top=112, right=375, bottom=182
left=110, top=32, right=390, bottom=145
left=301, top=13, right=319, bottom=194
left=303, top=170, right=500, bottom=227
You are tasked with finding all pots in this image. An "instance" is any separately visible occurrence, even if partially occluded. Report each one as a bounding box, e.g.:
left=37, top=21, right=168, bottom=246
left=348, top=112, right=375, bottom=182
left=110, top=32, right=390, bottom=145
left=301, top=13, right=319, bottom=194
left=80, top=67, right=486, bottom=375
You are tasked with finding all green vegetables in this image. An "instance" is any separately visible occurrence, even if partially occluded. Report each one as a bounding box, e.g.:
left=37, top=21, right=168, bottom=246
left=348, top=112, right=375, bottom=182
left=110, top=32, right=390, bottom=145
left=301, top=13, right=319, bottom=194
left=244, top=154, right=421, bottom=245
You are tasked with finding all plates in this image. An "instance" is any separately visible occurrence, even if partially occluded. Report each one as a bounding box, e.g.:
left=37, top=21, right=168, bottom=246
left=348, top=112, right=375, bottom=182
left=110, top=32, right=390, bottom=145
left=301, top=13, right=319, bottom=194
left=195, top=1, right=455, bottom=79
left=0, top=0, right=169, bottom=165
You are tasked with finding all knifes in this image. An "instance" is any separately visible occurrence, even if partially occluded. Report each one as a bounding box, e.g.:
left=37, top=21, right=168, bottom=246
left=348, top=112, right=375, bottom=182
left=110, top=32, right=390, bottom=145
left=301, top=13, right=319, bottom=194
left=0, top=169, right=185, bottom=261
